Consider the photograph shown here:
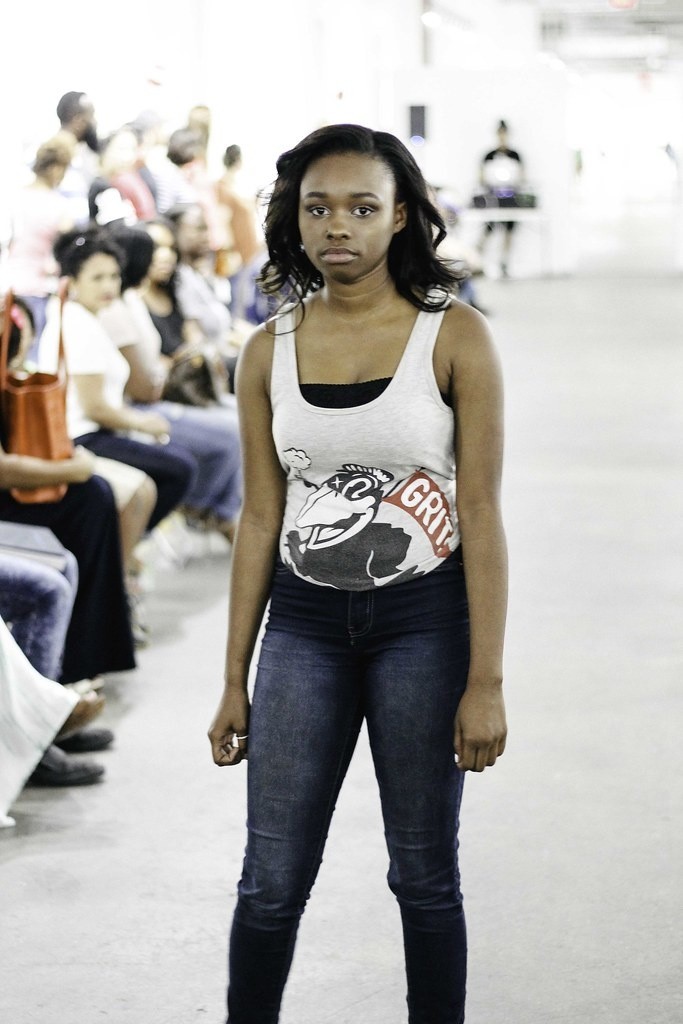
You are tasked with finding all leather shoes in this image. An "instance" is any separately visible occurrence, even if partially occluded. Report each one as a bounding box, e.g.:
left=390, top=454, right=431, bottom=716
left=25, top=742, right=105, bottom=788
left=49, top=728, right=114, bottom=754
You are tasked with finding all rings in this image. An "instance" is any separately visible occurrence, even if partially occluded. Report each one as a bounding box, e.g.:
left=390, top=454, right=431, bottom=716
left=236, top=736, right=247, bottom=740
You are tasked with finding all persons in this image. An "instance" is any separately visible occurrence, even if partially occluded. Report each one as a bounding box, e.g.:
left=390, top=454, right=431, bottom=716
left=208, top=124, right=512, bottom=1024
left=1, top=90, right=294, bottom=828
left=472, top=120, right=522, bottom=283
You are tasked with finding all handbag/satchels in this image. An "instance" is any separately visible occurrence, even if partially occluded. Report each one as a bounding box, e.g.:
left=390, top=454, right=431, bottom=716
left=9, top=372, right=73, bottom=504
left=162, top=352, right=222, bottom=410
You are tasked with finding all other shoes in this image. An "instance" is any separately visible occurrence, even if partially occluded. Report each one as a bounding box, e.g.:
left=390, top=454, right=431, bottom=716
left=216, top=518, right=235, bottom=546
left=63, top=678, right=108, bottom=729
left=129, top=605, right=154, bottom=649
